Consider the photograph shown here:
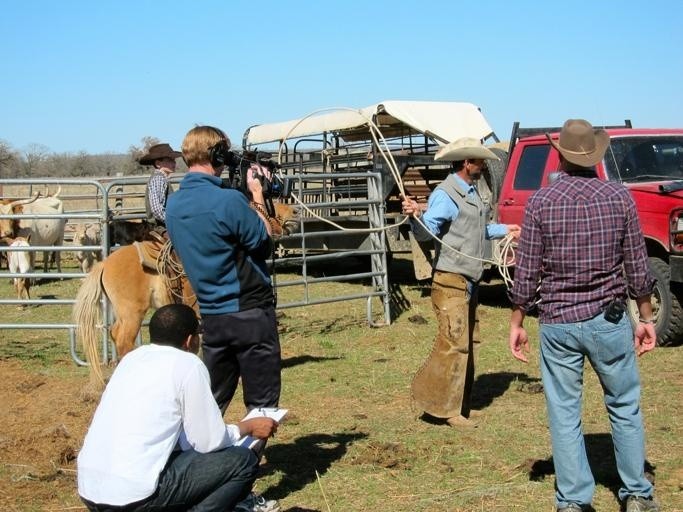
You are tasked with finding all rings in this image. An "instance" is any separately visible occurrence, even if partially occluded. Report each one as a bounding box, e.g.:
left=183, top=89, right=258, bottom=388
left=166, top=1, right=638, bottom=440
left=403, top=206, right=406, bottom=210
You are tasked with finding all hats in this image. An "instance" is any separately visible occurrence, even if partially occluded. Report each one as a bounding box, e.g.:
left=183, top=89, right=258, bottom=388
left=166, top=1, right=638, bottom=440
left=432, top=135, right=502, bottom=163
left=138, top=142, right=183, bottom=166
left=541, top=116, right=613, bottom=169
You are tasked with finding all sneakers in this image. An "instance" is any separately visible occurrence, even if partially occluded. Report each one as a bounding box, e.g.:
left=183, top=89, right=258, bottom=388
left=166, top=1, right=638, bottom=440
left=445, top=416, right=478, bottom=429
left=234, top=492, right=281, bottom=512
left=556, top=503, right=583, bottom=512
left=468, top=409, right=483, bottom=418
left=624, top=495, right=658, bottom=512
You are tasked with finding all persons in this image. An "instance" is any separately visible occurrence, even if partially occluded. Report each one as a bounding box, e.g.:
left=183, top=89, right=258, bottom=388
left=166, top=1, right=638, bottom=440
left=163, top=126, right=280, bottom=510
left=139, top=143, right=185, bottom=244
left=397, top=135, right=522, bottom=428
left=74, top=303, right=280, bottom=511
left=507, top=119, right=663, bottom=512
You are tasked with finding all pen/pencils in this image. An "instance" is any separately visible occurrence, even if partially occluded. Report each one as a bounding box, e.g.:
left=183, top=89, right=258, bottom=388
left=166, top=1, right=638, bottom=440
left=262, top=408, right=277, bottom=439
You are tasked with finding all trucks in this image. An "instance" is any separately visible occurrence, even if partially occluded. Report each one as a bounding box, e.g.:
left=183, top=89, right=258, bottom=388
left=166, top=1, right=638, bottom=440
left=497, top=127, right=683, bottom=348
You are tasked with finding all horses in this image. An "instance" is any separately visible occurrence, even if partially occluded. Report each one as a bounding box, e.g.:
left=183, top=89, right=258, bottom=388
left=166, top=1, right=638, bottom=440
left=70, top=199, right=301, bottom=387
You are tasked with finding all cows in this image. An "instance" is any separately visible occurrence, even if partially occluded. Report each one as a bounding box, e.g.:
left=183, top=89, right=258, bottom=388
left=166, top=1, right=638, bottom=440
left=0, top=184, right=166, bottom=311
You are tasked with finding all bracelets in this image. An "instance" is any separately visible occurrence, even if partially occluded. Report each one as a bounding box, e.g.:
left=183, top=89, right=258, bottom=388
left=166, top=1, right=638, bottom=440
left=637, top=316, right=656, bottom=325
